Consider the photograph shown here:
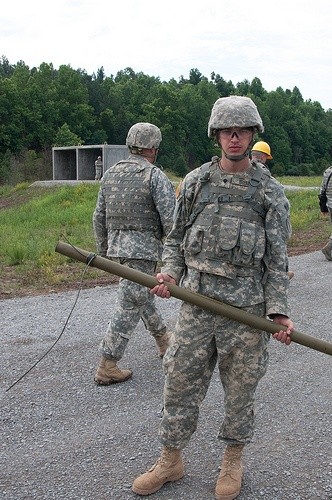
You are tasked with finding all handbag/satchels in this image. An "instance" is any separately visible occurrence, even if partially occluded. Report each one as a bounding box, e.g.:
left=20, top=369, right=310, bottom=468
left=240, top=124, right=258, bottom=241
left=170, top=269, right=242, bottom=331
left=318, top=172, right=332, bottom=214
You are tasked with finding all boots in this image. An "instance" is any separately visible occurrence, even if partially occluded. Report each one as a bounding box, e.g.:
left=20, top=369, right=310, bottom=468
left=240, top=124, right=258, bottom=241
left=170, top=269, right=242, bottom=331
left=129, top=447, right=191, bottom=497
left=215, top=442, right=245, bottom=500
left=154, top=330, right=174, bottom=361
left=95, top=355, right=133, bottom=384
left=321, top=238, right=332, bottom=261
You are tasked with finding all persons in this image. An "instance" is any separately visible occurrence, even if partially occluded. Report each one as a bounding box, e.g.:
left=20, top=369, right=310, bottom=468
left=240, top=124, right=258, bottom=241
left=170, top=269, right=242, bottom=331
left=321, top=166, right=332, bottom=261
left=132, top=97, right=294, bottom=500
left=250, top=141, right=294, bottom=280
left=95, top=156, right=103, bottom=180
left=91, top=123, right=177, bottom=386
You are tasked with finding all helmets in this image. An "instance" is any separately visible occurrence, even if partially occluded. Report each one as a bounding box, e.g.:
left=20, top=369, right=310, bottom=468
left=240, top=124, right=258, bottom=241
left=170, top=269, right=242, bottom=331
left=126, top=121, right=162, bottom=152
left=251, top=141, right=272, bottom=160
left=207, top=93, right=264, bottom=127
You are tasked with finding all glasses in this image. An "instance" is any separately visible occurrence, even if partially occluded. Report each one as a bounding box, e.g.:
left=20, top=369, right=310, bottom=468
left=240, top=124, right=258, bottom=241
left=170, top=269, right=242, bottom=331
left=218, top=127, right=252, bottom=141
